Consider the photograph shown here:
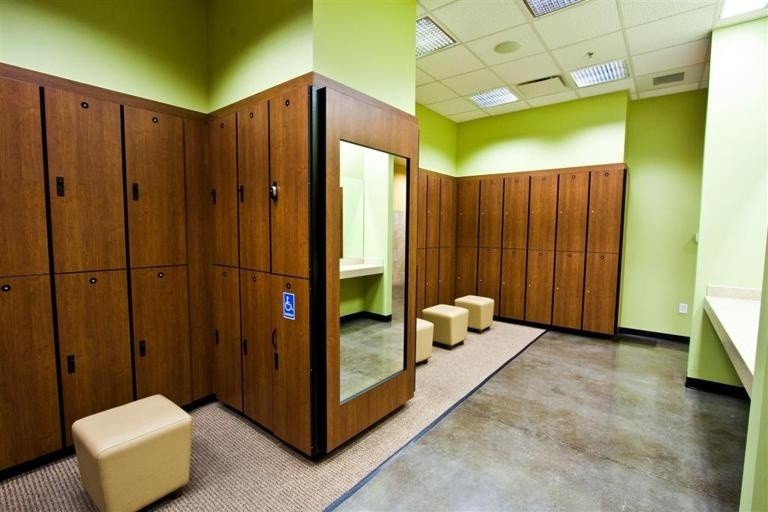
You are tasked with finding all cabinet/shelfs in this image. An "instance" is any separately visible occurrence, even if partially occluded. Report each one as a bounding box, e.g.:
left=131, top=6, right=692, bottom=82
left=416, top=161, right=629, bottom=337
left=209, top=70, right=315, bottom=462
left=0, top=60, right=208, bottom=491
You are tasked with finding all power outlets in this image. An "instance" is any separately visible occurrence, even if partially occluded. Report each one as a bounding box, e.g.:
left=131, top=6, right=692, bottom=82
left=679, top=303, right=688, bottom=314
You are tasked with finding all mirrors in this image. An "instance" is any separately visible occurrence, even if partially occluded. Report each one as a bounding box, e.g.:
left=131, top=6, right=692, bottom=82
left=338, top=137, right=411, bottom=406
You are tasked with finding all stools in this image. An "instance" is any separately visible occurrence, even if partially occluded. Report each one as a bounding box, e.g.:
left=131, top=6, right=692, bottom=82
left=414, top=302, right=469, bottom=367
left=71, top=393, right=194, bottom=512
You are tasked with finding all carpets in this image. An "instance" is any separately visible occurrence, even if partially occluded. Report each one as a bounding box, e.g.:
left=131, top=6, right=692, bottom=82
left=0, top=318, right=548, bottom=511
